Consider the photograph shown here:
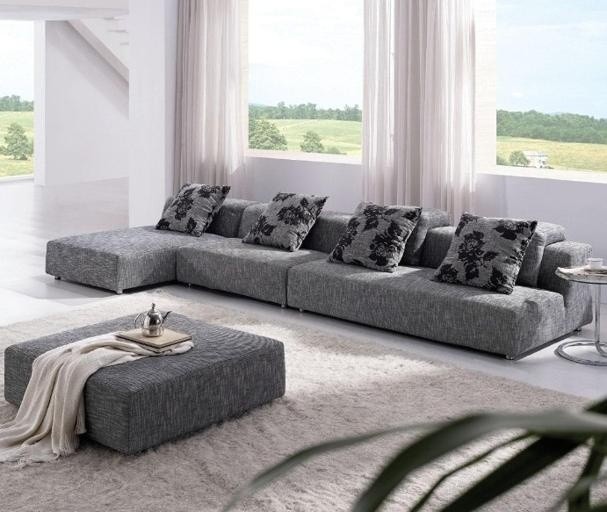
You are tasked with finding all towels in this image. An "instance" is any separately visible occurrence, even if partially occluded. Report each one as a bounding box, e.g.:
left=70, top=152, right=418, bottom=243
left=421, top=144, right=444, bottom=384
left=0, top=328, right=194, bottom=465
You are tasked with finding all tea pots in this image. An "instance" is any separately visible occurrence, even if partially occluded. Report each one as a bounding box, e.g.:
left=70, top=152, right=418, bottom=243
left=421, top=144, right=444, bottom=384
left=133, top=302, right=172, bottom=339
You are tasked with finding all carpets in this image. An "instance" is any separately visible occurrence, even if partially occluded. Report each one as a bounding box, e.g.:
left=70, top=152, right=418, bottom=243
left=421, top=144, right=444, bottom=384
left=0, top=286, right=607, bottom=512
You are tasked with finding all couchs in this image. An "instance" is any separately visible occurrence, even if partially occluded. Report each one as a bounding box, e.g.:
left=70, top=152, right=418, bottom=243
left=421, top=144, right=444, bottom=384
left=176, top=205, right=356, bottom=309
left=45, top=194, right=258, bottom=294
left=286, top=209, right=592, bottom=360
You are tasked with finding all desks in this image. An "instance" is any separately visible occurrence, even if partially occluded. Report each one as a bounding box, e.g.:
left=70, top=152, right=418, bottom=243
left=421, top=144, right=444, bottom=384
left=555, top=265, right=607, bottom=365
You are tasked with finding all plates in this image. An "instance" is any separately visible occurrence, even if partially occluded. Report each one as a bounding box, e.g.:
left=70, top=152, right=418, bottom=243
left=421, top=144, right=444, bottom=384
left=582, top=267, right=606, bottom=274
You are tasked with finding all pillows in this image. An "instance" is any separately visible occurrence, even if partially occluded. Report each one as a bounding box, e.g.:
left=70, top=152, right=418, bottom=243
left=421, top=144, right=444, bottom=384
left=241, top=190, right=330, bottom=252
left=429, top=212, right=538, bottom=294
left=327, top=201, right=423, bottom=272
left=154, top=180, right=231, bottom=235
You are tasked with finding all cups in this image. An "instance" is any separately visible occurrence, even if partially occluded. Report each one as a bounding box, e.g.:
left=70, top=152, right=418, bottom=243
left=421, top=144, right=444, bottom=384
left=585, top=258, right=603, bottom=270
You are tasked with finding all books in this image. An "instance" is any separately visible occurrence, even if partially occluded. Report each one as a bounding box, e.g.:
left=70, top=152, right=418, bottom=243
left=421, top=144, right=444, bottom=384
left=114, top=337, right=179, bottom=353
